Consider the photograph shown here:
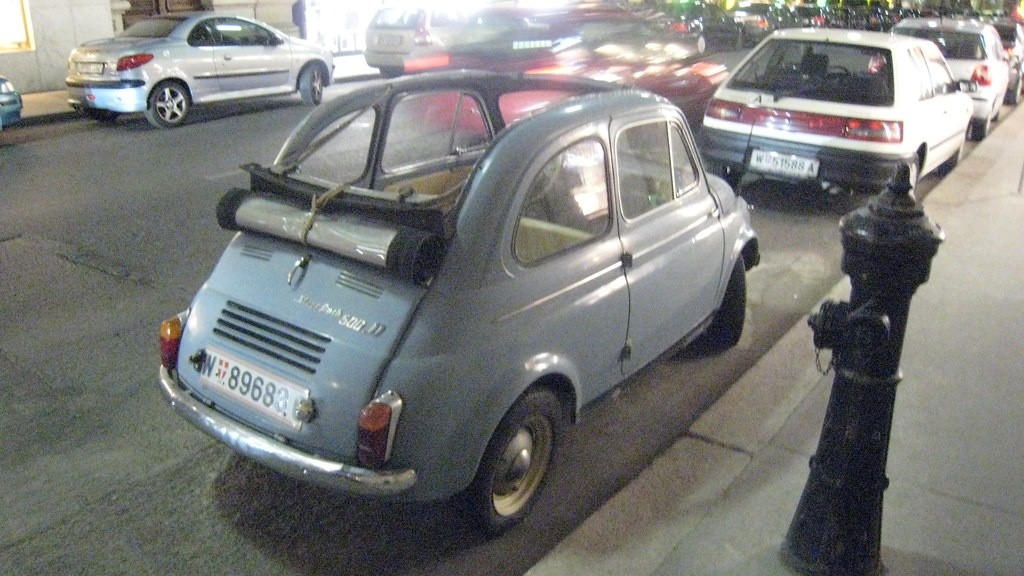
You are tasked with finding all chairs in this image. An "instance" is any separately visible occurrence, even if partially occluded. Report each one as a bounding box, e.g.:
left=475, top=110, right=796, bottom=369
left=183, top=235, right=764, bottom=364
left=801, top=53, right=830, bottom=80
left=947, top=41, right=981, bottom=58
left=850, top=75, right=891, bottom=98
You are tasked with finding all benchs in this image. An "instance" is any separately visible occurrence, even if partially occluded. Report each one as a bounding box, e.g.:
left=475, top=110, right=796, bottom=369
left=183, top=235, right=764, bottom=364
left=415, top=194, right=593, bottom=260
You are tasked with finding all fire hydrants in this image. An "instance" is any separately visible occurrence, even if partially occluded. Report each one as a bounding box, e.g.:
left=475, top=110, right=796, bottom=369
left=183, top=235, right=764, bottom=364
left=773, top=161, right=948, bottom=574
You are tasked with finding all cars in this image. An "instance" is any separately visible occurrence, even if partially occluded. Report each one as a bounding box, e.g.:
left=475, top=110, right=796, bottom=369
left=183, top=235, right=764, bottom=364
left=65, top=11, right=336, bottom=128
left=699, top=27, right=977, bottom=199
left=363, top=0, right=535, bottom=77
left=0, top=72, right=23, bottom=132
left=403, top=3, right=734, bottom=130
left=886, top=17, right=1019, bottom=139
left=553, top=0, right=1013, bottom=61
left=156, top=70, right=761, bottom=537
left=967, top=18, right=1024, bottom=106
